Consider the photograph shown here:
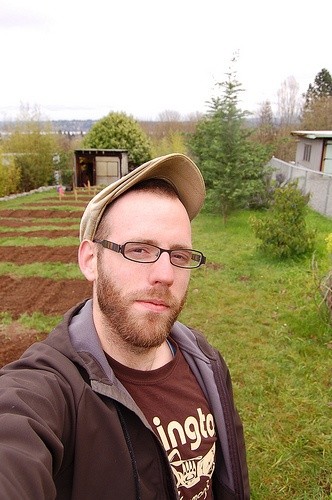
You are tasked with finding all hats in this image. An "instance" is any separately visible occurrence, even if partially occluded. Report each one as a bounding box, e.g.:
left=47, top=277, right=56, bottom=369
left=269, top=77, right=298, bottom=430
left=79, top=152, right=208, bottom=242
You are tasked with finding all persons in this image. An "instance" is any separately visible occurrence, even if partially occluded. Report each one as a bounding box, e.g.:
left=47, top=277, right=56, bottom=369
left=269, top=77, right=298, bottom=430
left=0, top=153, right=253, bottom=499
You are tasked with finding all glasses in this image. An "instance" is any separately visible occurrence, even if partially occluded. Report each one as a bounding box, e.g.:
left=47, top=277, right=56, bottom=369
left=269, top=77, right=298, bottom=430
left=92, top=239, right=206, bottom=269
